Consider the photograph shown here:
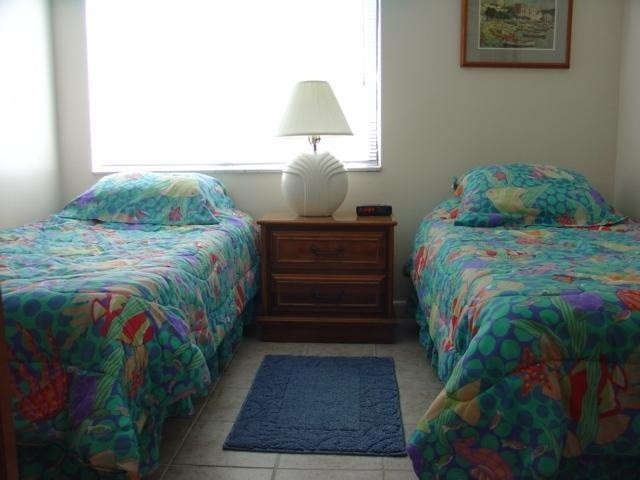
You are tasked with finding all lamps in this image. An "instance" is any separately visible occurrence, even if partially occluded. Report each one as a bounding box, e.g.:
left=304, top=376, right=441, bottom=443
left=274, top=80, right=354, bottom=218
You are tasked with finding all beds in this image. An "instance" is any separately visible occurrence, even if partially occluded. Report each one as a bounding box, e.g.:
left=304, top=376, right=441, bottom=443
left=0, top=209, right=260, bottom=480
left=402, top=195, right=640, bottom=479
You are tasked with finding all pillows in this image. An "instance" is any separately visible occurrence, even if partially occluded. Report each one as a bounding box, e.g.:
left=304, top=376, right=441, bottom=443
left=449, top=162, right=629, bottom=228
left=51, top=172, right=231, bottom=226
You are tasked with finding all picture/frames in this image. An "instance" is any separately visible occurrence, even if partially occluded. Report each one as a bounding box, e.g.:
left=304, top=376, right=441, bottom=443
left=459, top=0, right=573, bottom=69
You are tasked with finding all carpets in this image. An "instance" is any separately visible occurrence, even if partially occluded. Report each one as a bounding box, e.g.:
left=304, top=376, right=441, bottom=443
left=223, top=354, right=409, bottom=458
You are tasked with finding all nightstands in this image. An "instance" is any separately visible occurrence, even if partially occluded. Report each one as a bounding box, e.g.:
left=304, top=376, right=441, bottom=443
left=253, top=211, right=400, bottom=345
